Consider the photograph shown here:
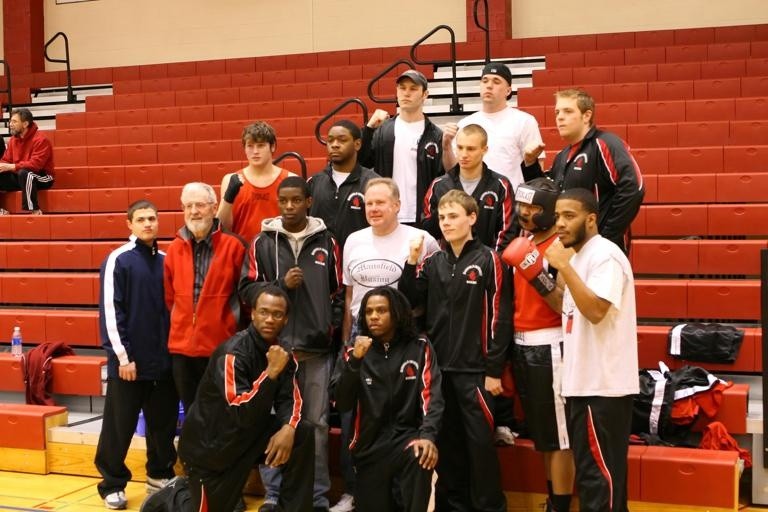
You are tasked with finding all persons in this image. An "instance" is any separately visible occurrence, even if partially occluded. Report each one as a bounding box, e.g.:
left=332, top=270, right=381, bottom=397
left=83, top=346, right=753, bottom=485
left=443, top=62, right=546, bottom=196
left=94, top=176, right=641, bottom=512
left=420, top=123, right=519, bottom=447
left=520, top=88, right=645, bottom=257
left=215, top=121, right=298, bottom=331
left=356, top=69, right=443, bottom=248
left=0, top=109, right=53, bottom=215
left=306, top=119, right=384, bottom=364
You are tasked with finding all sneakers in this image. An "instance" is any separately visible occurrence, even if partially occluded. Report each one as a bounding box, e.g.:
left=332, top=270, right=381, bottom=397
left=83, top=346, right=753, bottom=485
left=105, top=491, right=128, bottom=509
left=31, top=209, right=43, bottom=216
left=144, top=474, right=170, bottom=495
left=258, top=502, right=277, bottom=512
left=0, top=208, right=9, bottom=215
left=313, top=505, right=330, bottom=512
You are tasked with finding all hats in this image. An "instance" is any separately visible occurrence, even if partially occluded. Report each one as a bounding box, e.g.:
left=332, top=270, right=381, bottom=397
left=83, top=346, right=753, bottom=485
left=395, top=69, right=427, bottom=90
left=481, top=62, right=513, bottom=101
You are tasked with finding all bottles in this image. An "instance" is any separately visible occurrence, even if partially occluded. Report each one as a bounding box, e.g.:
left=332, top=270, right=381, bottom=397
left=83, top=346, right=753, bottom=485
left=12, top=327, right=23, bottom=362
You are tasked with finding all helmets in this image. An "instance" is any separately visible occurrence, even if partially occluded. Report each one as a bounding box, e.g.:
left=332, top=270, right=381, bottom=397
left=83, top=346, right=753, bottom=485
left=513, top=176, right=557, bottom=229
